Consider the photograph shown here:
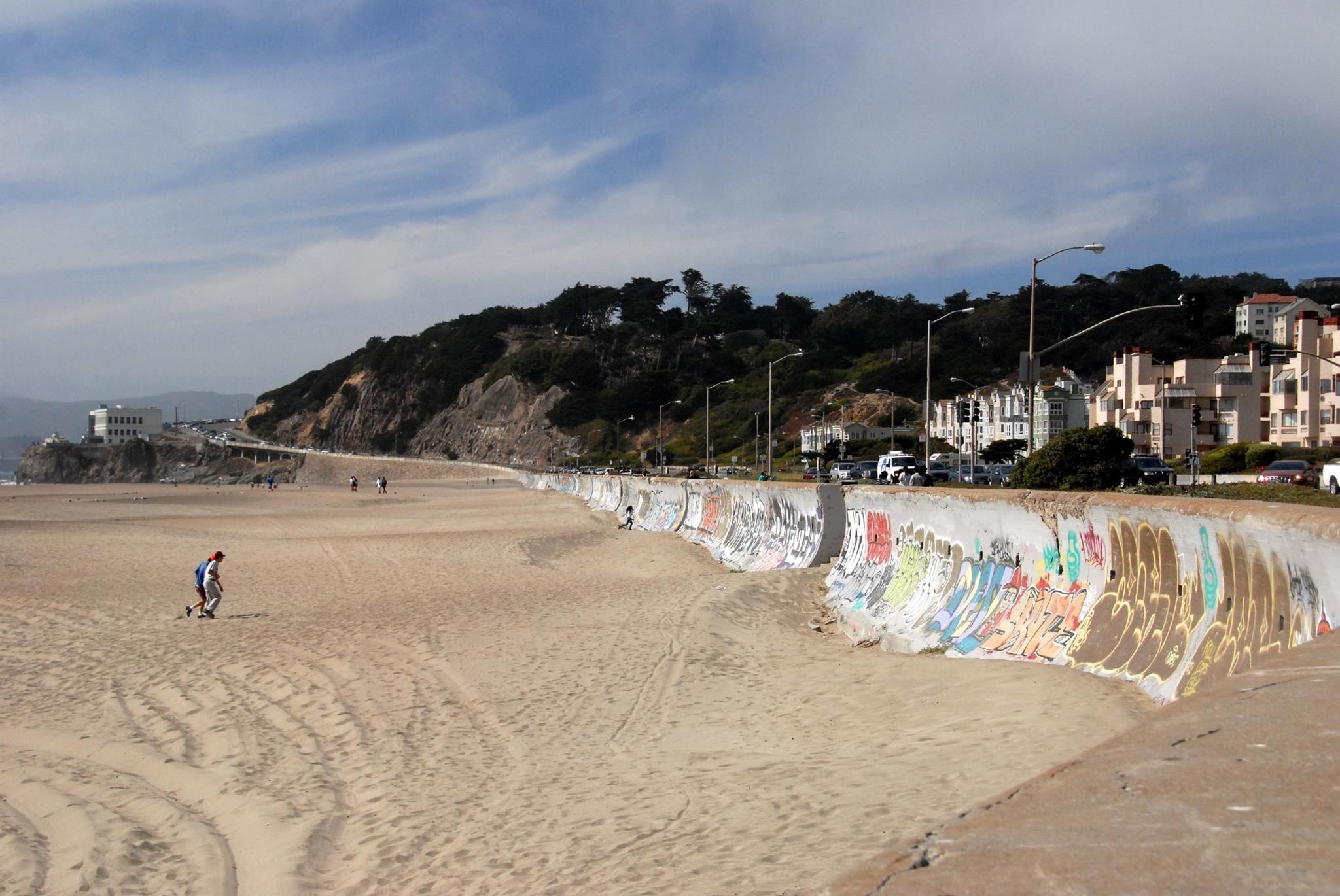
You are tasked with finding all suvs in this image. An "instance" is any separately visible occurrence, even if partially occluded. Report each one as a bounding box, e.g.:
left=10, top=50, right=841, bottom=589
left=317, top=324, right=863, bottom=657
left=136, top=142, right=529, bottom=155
left=830, top=462, right=856, bottom=481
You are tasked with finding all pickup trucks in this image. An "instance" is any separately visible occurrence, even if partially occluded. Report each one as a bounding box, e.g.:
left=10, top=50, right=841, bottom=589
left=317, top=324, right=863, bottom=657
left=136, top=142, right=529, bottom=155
left=1321, top=463, right=1340, bottom=495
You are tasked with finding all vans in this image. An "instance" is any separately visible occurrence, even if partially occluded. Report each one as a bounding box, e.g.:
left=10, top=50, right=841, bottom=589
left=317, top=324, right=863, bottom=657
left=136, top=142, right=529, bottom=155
left=877, top=450, right=918, bottom=485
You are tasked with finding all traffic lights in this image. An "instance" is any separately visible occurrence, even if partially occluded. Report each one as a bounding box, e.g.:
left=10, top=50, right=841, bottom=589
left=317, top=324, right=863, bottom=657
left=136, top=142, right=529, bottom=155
left=969, top=398, right=980, bottom=426
left=1192, top=404, right=1202, bottom=427
left=960, top=401, right=970, bottom=424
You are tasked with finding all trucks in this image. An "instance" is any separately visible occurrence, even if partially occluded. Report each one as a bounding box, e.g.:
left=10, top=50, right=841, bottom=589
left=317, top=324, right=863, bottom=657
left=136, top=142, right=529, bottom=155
left=929, top=452, right=985, bottom=469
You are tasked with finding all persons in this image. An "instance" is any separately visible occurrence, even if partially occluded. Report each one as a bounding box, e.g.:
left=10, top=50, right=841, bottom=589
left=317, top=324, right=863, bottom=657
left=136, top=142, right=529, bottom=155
left=898, top=466, right=911, bottom=486
left=376, top=477, right=382, bottom=493
left=921, top=465, right=933, bottom=486
left=909, top=466, right=924, bottom=486
left=758, top=471, right=768, bottom=481
left=349, top=476, right=358, bottom=492
left=382, top=477, right=387, bottom=494
left=642, top=466, right=650, bottom=477
left=605, top=469, right=611, bottom=475
left=202, top=551, right=225, bottom=619
left=619, top=505, right=634, bottom=530
left=185, top=554, right=215, bottom=618
left=875, top=471, right=890, bottom=485
left=267, top=476, right=274, bottom=489
left=687, top=469, right=699, bottom=479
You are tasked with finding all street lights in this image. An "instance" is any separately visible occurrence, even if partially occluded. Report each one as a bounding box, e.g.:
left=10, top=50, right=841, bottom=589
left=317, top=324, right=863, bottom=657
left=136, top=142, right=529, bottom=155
left=706, top=379, right=735, bottom=473
left=827, top=402, right=844, bottom=461
left=587, top=429, right=601, bottom=474
left=754, top=412, right=760, bottom=471
left=734, top=436, right=744, bottom=470
left=925, top=306, right=976, bottom=476
left=876, top=389, right=894, bottom=451
left=617, top=417, right=634, bottom=471
left=395, top=432, right=403, bottom=454
left=550, top=435, right=581, bottom=472
left=950, top=377, right=978, bottom=463
left=660, top=401, right=682, bottom=473
left=1131, top=352, right=1165, bottom=462
left=782, top=431, right=795, bottom=474
left=703, top=437, right=713, bottom=462
left=757, top=433, right=773, bottom=473
left=766, top=349, right=805, bottom=478
left=810, top=408, right=828, bottom=456
left=1026, top=242, right=1107, bottom=457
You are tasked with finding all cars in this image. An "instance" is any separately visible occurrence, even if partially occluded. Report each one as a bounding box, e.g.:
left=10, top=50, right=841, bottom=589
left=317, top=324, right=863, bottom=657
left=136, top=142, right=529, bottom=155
left=987, top=464, right=1015, bottom=487
left=559, top=466, right=633, bottom=476
left=727, top=467, right=737, bottom=474
left=181, top=417, right=356, bottom=455
left=803, top=466, right=832, bottom=483
left=850, top=461, right=879, bottom=480
left=1128, top=456, right=1177, bottom=487
left=1256, top=460, right=1320, bottom=490
left=948, top=464, right=991, bottom=485
left=918, top=460, right=950, bottom=483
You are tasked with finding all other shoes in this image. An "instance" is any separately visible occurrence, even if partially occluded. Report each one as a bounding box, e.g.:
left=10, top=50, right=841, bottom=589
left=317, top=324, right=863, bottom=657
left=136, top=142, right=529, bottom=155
left=203, top=608, right=214, bottom=619
left=186, top=606, right=192, bottom=617
left=198, top=614, right=206, bottom=618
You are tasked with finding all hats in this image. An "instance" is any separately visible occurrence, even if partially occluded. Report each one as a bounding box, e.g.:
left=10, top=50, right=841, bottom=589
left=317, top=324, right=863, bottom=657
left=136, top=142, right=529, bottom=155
left=209, top=554, right=214, bottom=560
left=214, top=551, right=225, bottom=556
